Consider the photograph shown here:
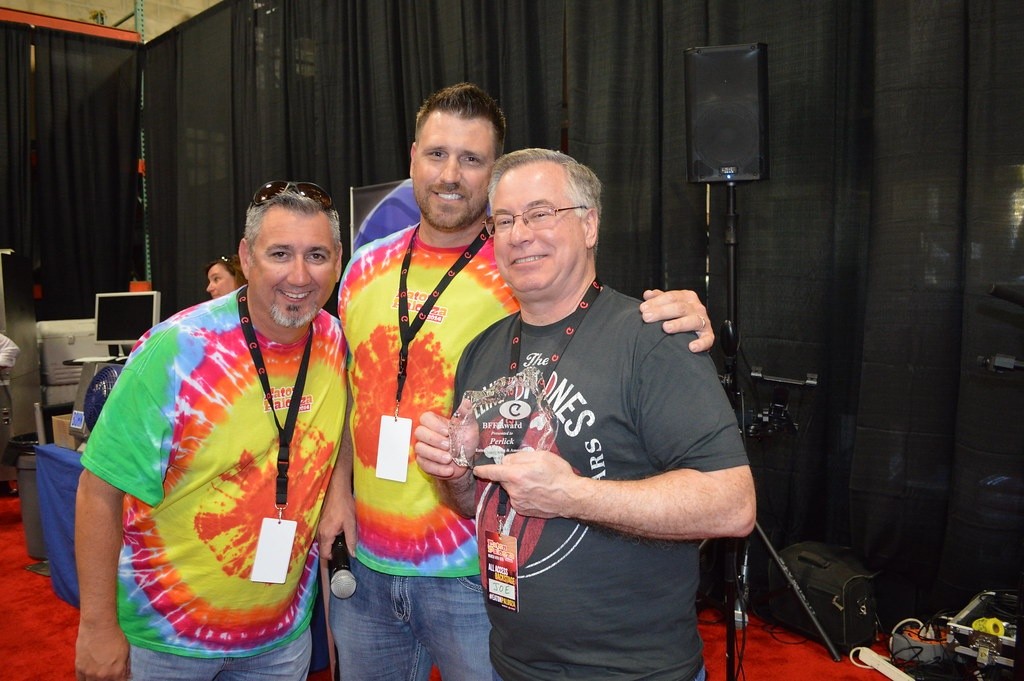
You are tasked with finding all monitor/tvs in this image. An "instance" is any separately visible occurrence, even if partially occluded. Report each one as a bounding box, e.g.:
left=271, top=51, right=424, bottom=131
left=94, top=291, right=161, bottom=345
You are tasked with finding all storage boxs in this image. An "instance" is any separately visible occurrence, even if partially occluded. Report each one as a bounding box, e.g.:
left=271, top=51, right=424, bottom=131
left=947, top=592, right=1016, bottom=667
left=52, top=414, right=83, bottom=451
left=36, top=319, right=110, bottom=385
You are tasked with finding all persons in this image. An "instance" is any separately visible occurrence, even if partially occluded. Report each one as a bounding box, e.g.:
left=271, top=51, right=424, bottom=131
left=72, top=183, right=347, bottom=680
left=0, top=332, right=21, bottom=497
left=414, top=148, right=758, bottom=681
left=206, top=255, right=245, bottom=298
left=319, top=81, right=716, bottom=680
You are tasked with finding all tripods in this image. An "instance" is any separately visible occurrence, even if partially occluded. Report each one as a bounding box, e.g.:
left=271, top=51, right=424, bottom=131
left=683, top=182, right=841, bottom=680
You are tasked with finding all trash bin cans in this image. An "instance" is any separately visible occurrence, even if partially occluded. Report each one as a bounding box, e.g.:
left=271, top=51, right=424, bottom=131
left=8, top=432, right=54, bottom=560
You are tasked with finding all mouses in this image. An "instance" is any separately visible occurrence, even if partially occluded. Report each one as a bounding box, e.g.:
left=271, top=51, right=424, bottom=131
left=115, top=356, right=129, bottom=361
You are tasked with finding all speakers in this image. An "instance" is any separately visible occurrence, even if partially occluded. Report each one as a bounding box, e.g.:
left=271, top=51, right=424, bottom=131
left=682, top=42, right=769, bottom=182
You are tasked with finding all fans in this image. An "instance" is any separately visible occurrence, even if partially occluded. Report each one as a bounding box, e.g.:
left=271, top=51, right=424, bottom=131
left=84, top=365, right=123, bottom=432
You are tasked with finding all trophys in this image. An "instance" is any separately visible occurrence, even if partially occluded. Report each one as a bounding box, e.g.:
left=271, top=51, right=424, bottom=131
left=448, top=366, right=560, bottom=470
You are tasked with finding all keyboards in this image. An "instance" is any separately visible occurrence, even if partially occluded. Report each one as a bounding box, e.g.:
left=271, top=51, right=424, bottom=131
left=72, top=356, right=117, bottom=363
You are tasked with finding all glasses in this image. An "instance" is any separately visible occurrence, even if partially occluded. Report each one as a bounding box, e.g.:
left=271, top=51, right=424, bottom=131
left=249, top=180, right=338, bottom=220
left=481, top=205, right=589, bottom=237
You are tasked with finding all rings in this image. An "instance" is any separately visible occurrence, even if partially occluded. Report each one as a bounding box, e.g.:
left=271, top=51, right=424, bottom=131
left=698, top=315, right=706, bottom=333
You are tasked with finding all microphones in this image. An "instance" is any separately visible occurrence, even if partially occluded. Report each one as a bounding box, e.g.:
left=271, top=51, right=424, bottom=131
left=330, top=531, right=356, bottom=599
